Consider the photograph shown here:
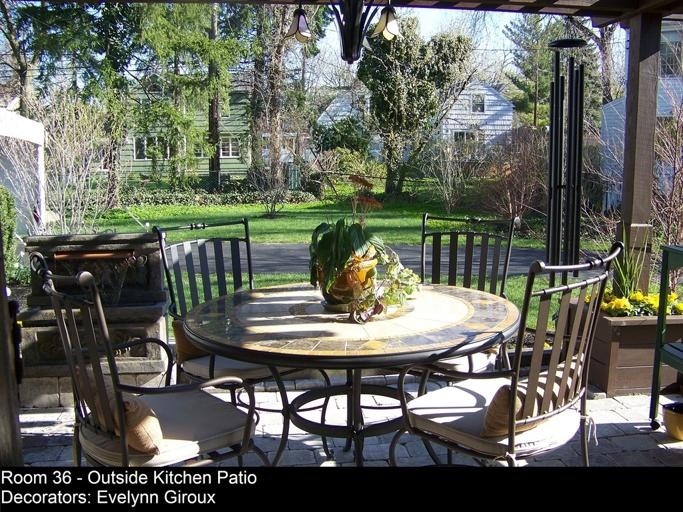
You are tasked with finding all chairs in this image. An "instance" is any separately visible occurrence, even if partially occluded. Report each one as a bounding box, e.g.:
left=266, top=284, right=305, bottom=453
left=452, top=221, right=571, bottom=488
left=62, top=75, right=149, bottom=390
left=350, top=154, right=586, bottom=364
left=29, top=213, right=626, bottom=467
